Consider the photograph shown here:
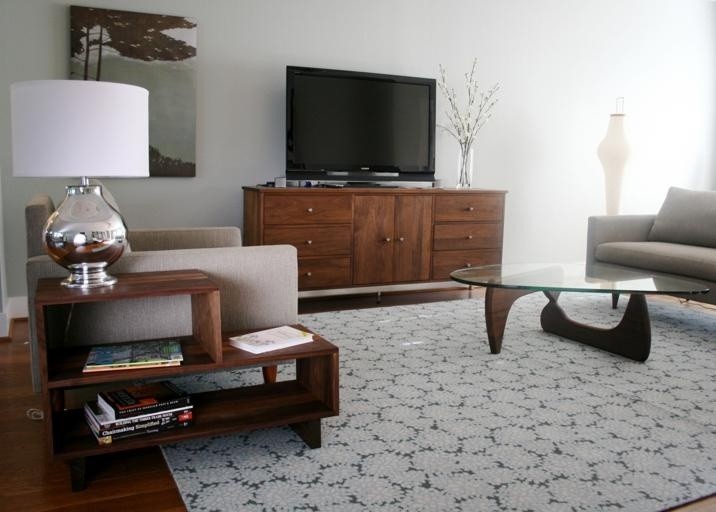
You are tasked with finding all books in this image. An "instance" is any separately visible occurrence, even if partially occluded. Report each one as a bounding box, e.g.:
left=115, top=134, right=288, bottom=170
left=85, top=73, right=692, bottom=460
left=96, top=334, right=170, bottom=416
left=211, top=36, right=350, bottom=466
left=84, top=379, right=193, bottom=444
left=82, top=361, right=182, bottom=372
left=228, top=325, right=314, bottom=354
left=85, top=339, right=183, bottom=368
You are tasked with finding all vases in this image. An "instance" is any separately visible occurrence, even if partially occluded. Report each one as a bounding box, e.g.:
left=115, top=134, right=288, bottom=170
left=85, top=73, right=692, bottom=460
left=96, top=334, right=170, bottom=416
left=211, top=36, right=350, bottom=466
left=457, top=144, right=474, bottom=190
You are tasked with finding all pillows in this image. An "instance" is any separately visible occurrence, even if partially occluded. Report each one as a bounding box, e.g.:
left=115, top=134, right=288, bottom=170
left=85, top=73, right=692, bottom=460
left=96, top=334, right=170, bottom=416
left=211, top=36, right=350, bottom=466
left=89, top=179, right=131, bottom=253
left=647, top=187, right=716, bottom=249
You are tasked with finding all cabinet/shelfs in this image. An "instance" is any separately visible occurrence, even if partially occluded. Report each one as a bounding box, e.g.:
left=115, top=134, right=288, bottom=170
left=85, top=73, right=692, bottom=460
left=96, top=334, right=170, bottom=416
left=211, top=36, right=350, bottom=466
left=34, top=270, right=339, bottom=493
left=241, top=185, right=509, bottom=296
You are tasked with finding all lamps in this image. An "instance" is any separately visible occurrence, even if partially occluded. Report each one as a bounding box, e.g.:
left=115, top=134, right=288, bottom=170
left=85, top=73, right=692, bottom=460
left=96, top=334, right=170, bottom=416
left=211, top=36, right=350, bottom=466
left=10, top=80, right=150, bottom=290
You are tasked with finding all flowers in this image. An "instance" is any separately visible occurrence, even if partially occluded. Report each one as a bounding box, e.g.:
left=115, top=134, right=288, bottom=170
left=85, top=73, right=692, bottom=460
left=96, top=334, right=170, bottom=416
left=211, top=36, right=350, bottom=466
left=435, top=56, right=501, bottom=186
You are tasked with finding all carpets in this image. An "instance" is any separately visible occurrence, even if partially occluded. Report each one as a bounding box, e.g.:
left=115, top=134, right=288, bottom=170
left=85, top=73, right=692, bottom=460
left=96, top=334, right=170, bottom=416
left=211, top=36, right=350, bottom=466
left=148, top=292, right=716, bottom=512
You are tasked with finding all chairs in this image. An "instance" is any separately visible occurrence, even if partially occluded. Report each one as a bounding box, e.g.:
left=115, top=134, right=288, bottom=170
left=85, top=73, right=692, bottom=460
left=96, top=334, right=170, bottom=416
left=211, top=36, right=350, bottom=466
left=25, top=194, right=299, bottom=392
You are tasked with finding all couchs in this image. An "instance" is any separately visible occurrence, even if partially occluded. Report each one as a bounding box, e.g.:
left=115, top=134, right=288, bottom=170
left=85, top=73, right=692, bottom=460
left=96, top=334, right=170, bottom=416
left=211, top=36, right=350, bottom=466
left=585, top=187, right=716, bottom=309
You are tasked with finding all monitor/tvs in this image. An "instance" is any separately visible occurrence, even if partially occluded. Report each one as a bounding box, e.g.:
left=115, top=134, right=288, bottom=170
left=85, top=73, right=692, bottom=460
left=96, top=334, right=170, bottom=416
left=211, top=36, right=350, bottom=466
left=285, top=65, right=436, bottom=188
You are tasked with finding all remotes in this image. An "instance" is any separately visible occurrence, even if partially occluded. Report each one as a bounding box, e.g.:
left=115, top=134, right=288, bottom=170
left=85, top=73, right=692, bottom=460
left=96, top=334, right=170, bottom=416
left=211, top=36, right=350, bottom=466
left=326, top=184, right=344, bottom=188
